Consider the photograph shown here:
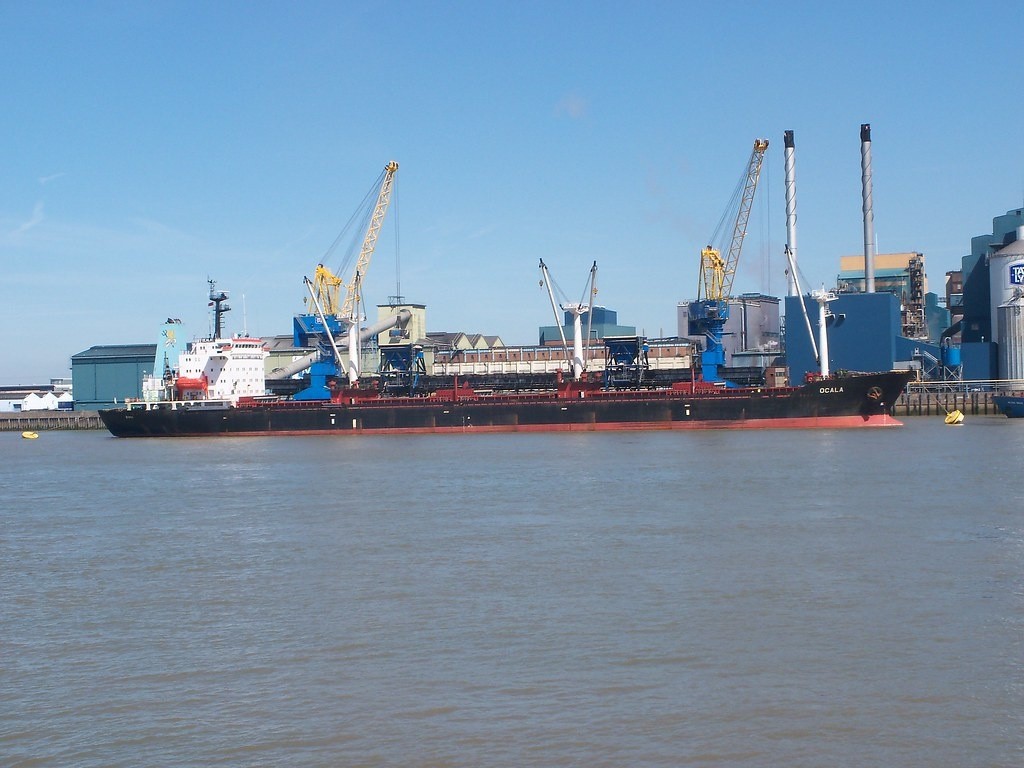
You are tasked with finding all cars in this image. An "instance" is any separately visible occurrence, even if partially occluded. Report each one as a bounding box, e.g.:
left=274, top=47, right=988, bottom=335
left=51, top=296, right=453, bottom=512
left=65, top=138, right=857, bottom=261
left=990, top=395, right=1024, bottom=417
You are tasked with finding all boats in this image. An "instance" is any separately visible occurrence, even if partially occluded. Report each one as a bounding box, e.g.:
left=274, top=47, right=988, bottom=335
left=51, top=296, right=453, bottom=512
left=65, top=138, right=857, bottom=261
left=94, top=245, right=926, bottom=436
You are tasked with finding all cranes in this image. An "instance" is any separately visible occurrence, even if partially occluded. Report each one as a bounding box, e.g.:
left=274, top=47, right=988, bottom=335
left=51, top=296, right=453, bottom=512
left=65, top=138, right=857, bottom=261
left=306, top=160, right=403, bottom=326
left=685, top=136, right=773, bottom=325
